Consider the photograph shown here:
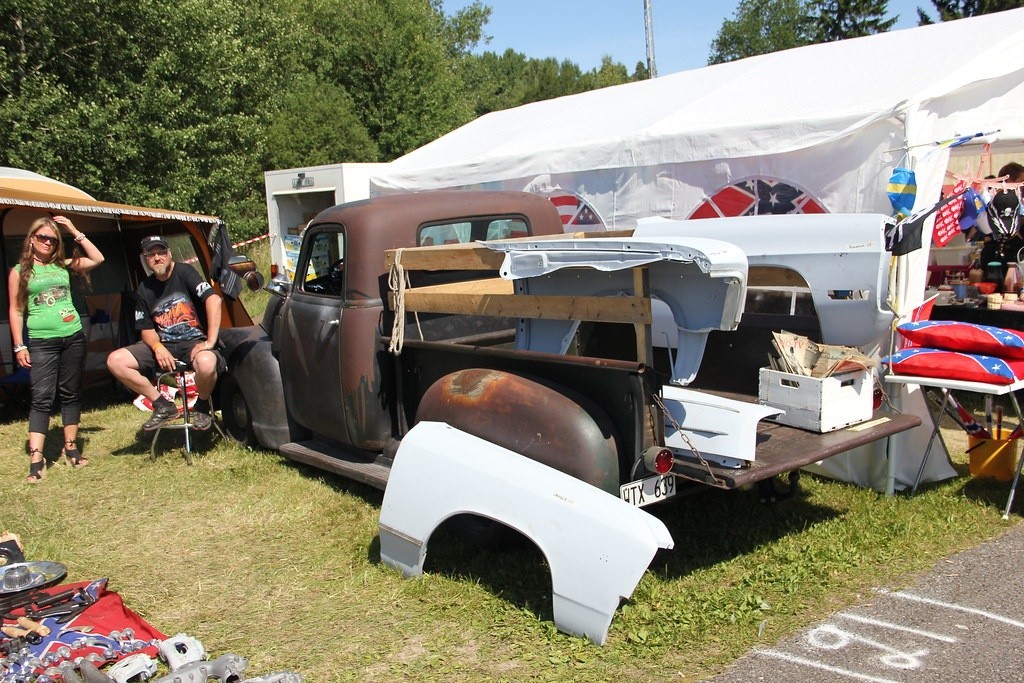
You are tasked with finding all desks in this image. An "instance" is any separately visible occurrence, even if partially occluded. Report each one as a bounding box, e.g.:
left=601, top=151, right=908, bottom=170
left=883, top=374, right=1024, bottom=520
left=932, top=303, right=1024, bottom=331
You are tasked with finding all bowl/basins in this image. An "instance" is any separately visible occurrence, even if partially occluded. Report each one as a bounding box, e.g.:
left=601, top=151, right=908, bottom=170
left=971, top=283, right=998, bottom=295
left=3, top=565, right=33, bottom=590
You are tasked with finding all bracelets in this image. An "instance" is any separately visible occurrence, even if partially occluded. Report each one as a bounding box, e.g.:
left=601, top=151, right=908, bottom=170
left=204, top=341, right=210, bottom=350
left=13, top=344, right=27, bottom=353
left=74, top=233, right=86, bottom=244
left=153, top=343, right=163, bottom=350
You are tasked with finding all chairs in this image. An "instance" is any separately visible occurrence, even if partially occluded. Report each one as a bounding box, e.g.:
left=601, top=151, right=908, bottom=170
left=76, top=290, right=125, bottom=403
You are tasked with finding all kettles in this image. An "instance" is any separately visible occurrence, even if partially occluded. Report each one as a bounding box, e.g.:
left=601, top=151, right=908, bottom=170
left=1004, top=262, right=1023, bottom=298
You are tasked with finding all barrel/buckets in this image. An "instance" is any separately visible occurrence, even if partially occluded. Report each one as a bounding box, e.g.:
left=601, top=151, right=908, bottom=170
left=968, top=427, right=1017, bottom=482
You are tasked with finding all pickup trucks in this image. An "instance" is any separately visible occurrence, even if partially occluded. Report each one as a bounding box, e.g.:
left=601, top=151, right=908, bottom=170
left=211, top=185, right=923, bottom=567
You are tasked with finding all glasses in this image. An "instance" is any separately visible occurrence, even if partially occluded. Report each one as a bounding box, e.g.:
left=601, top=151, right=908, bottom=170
left=144, top=249, right=171, bottom=259
left=34, top=234, right=58, bottom=246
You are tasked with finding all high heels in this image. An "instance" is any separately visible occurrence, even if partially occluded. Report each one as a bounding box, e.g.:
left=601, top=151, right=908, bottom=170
left=28, top=457, right=47, bottom=484
left=62, top=447, right=88, bottom=469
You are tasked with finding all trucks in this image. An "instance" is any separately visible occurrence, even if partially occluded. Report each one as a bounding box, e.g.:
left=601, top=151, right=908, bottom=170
left=0, top=166, right=259, bottom=417
left=264, top=163, right=391, bottom=291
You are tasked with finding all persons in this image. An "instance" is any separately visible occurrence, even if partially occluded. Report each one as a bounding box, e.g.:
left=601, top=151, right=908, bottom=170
left=9, top=216, right=104, bottom=482
left=967, top=162, right=1024, bottom=241
left=106, top=235, right=222, bottom=431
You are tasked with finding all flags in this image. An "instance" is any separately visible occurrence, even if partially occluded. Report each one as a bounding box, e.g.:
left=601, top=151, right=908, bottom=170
left=1006, top=423, right=1024, bottom=439
left=900, top=295, right=936, bottom=349
left=948, top=391, right=992, bottom=439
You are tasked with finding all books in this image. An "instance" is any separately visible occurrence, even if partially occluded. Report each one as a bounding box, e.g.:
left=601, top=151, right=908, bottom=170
left=768, top=330, right=880, bottom=387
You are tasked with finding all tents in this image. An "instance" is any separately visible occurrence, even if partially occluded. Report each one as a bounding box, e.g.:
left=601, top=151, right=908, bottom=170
left=0, top=166, right=254, bottom=385
left=369, top=5, right=1024, bottom=494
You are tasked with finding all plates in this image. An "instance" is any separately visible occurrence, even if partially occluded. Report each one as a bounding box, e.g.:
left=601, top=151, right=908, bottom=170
left=0, top=561, right=67, bottom=594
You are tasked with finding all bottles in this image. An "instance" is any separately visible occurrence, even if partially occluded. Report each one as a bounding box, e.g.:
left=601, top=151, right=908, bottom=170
left=969, top=258, right=984, bottom=284
left=988, top=262, right=1006, bottom=294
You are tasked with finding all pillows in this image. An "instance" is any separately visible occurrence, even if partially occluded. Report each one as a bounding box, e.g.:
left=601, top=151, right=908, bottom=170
left=895, top=319, right=1024, bottom=361
left=881, top=348, right=1024, bottom=386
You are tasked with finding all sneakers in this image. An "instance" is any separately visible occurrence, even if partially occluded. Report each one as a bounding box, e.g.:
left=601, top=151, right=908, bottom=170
left=194, top=400, right=212, bottom=430
left=142, top=402, right=178, bottom=430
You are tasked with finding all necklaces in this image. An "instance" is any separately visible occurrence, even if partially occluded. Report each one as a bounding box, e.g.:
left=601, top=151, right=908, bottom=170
left=34, top=258, right=50, bottom=263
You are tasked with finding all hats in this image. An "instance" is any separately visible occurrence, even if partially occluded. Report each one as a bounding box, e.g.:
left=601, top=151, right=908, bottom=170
left=141, top=236, right=169, bottom=252
left=958, top=186, right=1020, bottom=238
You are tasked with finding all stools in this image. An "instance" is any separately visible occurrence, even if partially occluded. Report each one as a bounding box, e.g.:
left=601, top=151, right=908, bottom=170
left=149, top=365, right=212, bottom=465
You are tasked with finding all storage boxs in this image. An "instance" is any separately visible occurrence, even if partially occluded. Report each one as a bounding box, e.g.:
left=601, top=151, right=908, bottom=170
left=758, top=368, right=874, bottom=433
left=928, top=245, right=978, bottom=266
left=284, top=212, right=338, bottom=284
